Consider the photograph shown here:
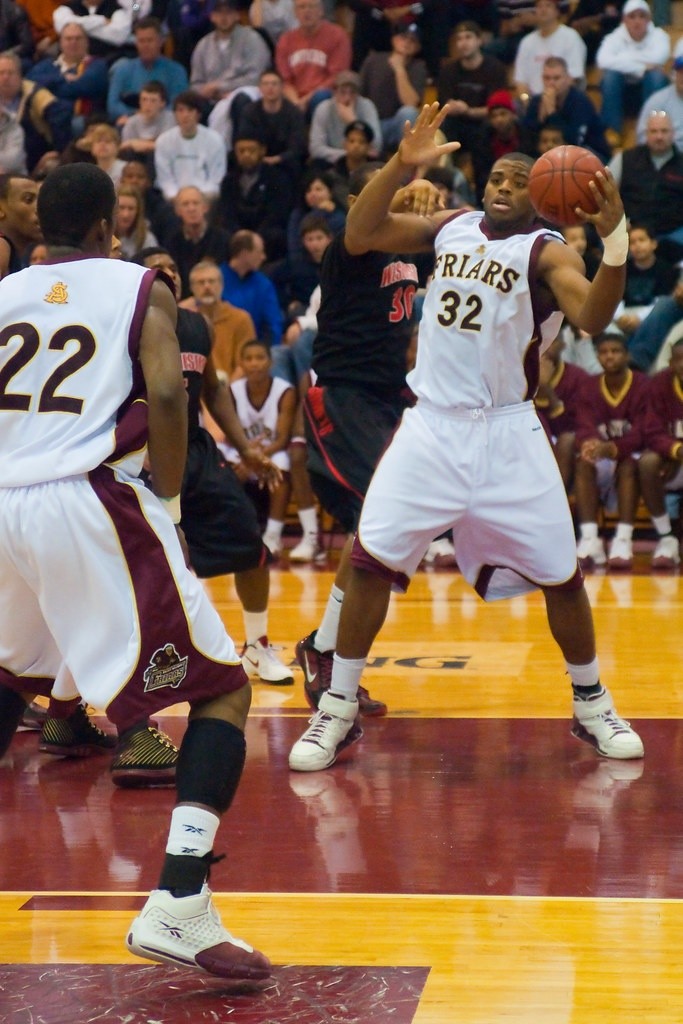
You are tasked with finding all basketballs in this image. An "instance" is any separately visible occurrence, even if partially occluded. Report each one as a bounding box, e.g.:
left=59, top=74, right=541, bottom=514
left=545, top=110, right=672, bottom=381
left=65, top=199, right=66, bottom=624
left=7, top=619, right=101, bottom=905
left=528, top=145, right=607, bottom=227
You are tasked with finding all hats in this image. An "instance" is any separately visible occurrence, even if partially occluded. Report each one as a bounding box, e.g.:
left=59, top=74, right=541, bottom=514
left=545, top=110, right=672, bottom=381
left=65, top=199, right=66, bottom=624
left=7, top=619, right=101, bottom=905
left=335, top=70, right=363, bottom=95
left=486, top=91, right=517, bottom=117
left=455, top=21, right=480, bottom=41
left=395, top=22, right=420, bottom=41
left=624, top=1, right=653, bottom=18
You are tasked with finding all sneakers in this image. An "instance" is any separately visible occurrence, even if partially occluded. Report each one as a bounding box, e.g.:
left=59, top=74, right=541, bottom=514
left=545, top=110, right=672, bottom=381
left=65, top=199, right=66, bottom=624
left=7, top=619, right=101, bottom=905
left=110, top=727, right=182, bottom=786
left=291, top=536, right=320, bottom=561
left=239, top=638, right=294, bottom=685
left=652, top=538, right=678, bottom=567
left=570, top=755, right=644, bottom=807
left=292, top=773, right=361, bottom=838
left=288, top=688, right=366, bottom=772
left=294, top=635, right=388, bottom=717
left=37, top=713, right=117, bottom=758
left=127, top=883, right=270, bottom=980
left=574, top=535, right=606, bottom=568
left=609, top=534, right=634, bottom=566
left=422, top=540, right=455, bottom=565
left=264, top=537, right=284, bottom=559
left=16, top=703, right=47, bottom=729
left=569, top=689, right=644, bottom=759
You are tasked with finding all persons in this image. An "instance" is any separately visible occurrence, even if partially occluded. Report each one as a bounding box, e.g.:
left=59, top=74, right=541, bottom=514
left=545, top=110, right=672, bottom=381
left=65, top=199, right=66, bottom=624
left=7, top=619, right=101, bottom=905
left=1, top=0, right=682, bottom=788
left=0, top=160, right=271, bottom=988
